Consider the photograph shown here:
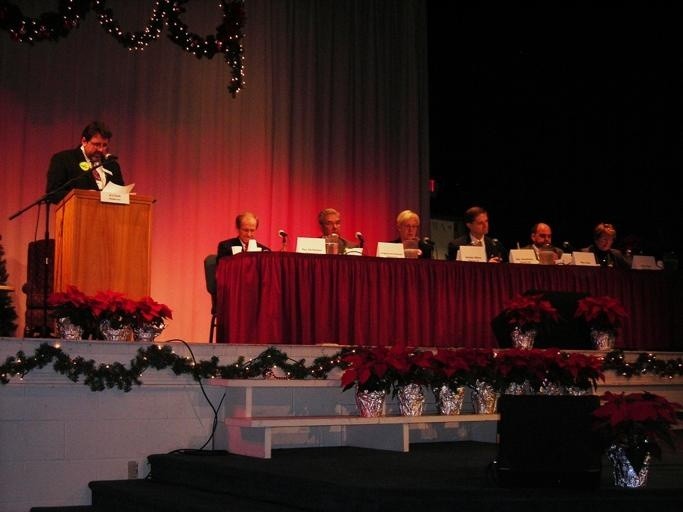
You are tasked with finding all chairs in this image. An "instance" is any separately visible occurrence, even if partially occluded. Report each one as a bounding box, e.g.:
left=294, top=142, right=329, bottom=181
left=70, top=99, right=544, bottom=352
left=203, top=254, right=218, bottom=343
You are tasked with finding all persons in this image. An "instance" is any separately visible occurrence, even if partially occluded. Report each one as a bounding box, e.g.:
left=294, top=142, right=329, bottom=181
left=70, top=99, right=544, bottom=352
left=318, top=208, right=360, bottom=255
left=449, top=207, right=508, bottom=263
left=518, top=223, right=565, bottom=265
left=586, top=223, right=623, bottom=264
left=46, top=122, right=124, bottom=204
left=391, top=210, right=431, bottom=259
left=216, top=212, right=271, bottom=265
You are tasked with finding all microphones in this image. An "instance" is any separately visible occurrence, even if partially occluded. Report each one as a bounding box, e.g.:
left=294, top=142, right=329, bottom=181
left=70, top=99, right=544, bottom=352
left=105, top=153, right=120, bottom=162
left=563, top=241, right=575, bottom=252
left=355, top=231, right=365, bottom=242
left=626, top=249, right=635, bottom=257
left=278, top=230, right=288, bottom=238
left=423, top=237, right=436, bottom=246
left=492, top=239, right=506, bottom=253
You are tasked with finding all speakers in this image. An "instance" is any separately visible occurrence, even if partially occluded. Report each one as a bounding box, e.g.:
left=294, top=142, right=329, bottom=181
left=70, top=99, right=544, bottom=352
left=498, top=392, right=601, bottom=490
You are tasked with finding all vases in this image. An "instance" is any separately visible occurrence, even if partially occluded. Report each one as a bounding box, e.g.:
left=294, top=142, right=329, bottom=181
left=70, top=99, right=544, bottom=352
left=353, top=379, right=593, bottom=419
left=54, top=311, right=87, bottom=342
left=510, top=318, right=537, bottom=351
left=130, top=314, right=164, bottom=343
left=97, top=316, right=132, bottom=341
left=605, top=435, right=651, bottom=490
left=585, top=322, right=617, bottom=353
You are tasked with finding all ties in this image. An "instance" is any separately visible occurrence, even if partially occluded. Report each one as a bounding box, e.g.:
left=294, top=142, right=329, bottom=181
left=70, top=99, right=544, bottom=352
left=90, top=161, right=101, bottom=180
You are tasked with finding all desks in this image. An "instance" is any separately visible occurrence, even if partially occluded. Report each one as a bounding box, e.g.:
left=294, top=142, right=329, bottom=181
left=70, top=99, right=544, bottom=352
left=220, top=251, right=674, bottom=348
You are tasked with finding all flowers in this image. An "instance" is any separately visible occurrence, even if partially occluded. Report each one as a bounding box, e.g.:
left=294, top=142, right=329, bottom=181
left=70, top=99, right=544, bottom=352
left=338, top=345, right=607, bottom=393
left=498, top=291, right=557, bottom=332
left=89, top=288, right=137, bottom=320
left=575, top=294, right=634, bottom=330
left=129, top=292, right=175, bottom=323
left=590, top=390, right=682, bottom=453
left=49, top=284, right=93, bottom=325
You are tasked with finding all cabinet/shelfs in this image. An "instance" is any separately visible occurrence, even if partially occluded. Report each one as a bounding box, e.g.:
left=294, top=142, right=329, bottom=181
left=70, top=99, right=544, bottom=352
left=206, top=378, right=502, bottom=461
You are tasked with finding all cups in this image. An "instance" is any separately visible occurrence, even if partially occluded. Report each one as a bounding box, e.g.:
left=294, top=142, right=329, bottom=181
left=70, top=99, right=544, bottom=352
left=403, top=240, right=418, bottom=258
left=326, top=238, right=338, bottom=254
left=248, top=240, right=257, bottom=251
left=664, top=256, right=673, bottom=271
left=539, top=251, right=553, bottom=265
left=604, top=252, right=617, bottom=267
left=231, top=246, right=242, bottom=255
